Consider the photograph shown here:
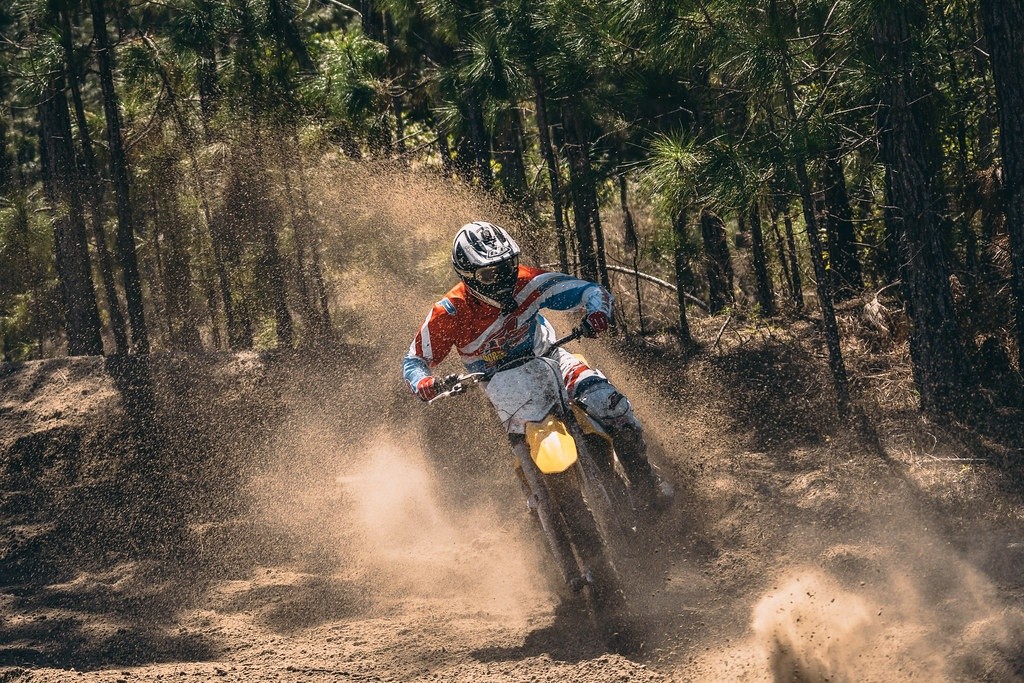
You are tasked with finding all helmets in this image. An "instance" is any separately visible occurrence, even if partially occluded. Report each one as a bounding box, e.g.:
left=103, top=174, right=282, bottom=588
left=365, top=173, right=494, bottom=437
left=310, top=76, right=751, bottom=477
left=450, top=221, right=521, bottom=312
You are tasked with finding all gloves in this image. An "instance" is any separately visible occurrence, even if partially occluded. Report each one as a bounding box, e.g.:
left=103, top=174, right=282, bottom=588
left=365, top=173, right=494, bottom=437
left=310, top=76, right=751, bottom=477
left=416, top=376, right=443, bottom=401
left=578, top=312, right=609, bottom=340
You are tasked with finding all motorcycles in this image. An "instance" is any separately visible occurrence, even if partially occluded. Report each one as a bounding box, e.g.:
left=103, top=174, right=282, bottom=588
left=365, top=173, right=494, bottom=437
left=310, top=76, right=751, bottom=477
left=428, top=318, right=645, bottom=657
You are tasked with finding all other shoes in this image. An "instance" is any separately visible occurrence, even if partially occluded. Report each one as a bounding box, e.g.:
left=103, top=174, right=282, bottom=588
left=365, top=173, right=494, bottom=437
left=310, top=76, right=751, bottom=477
left=630, top=466, right=675, bottom=511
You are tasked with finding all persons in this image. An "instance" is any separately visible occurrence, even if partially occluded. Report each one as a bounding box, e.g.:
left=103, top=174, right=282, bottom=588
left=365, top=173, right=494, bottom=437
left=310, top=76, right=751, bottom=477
left=401, top=220, right=678, bottom=533
